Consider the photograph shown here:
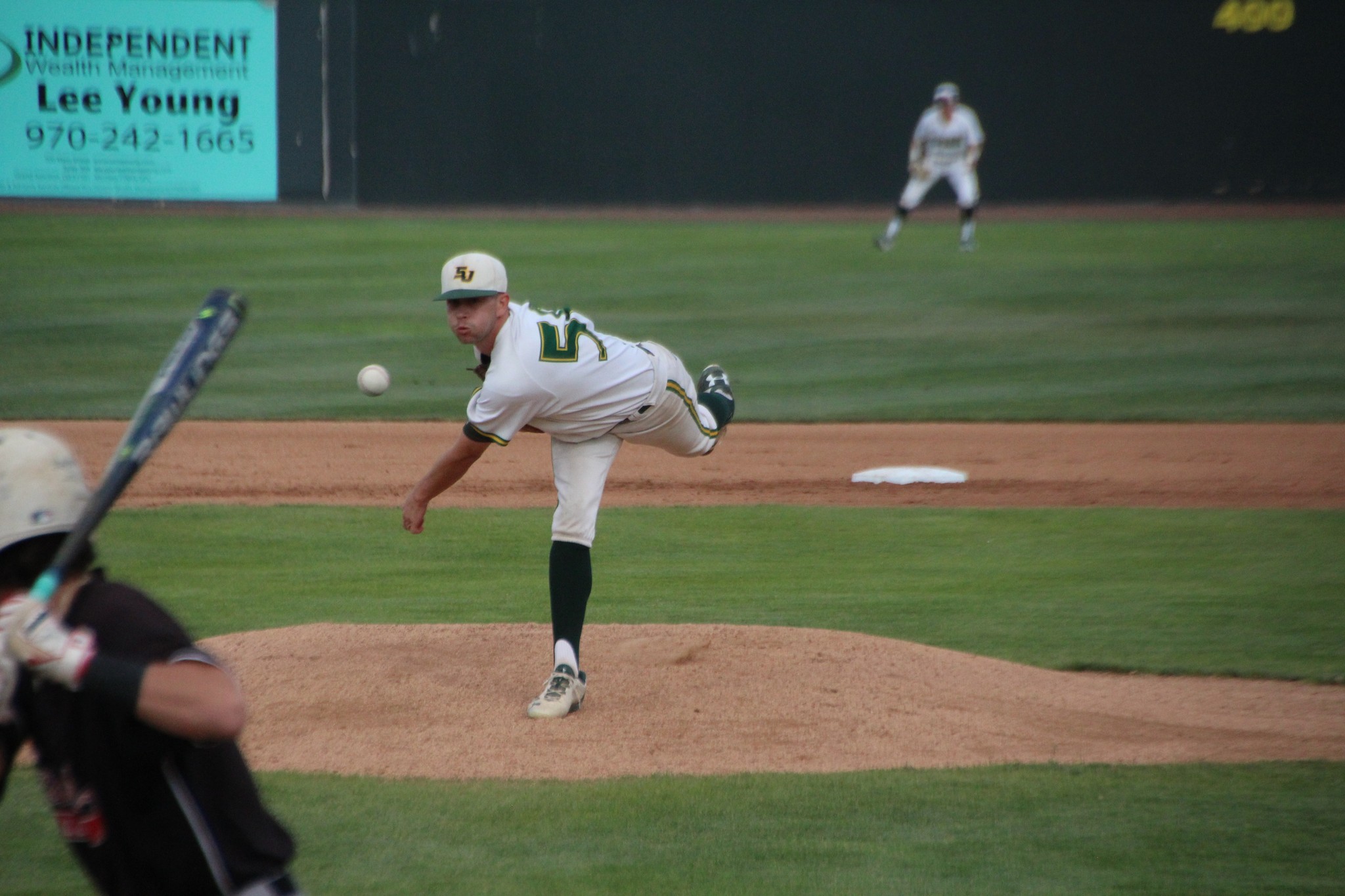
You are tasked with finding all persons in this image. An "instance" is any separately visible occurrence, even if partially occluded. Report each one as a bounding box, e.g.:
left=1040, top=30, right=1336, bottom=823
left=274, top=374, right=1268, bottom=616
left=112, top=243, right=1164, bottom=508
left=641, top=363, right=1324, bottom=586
left=402, top=252, right=735, bottom=720
left=0, top=429, right=298, bottom=896
left=872, top=81, right=988, bottom=255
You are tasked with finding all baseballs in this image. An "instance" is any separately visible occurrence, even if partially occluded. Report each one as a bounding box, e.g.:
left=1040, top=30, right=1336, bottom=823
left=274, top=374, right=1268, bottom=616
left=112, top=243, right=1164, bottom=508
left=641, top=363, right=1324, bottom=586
left=357, top=361, right=391, bottom=398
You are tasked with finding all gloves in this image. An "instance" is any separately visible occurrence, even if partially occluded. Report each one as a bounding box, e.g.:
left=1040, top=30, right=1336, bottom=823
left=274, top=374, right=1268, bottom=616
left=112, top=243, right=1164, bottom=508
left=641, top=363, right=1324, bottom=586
left=0, top=594, right=96, bottom=691
left=0, top=651, right=17, bottom=722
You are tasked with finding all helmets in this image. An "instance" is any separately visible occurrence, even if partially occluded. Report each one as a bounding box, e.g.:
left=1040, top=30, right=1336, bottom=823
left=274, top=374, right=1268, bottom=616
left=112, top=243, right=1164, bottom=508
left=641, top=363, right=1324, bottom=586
left=0, top=429, right=92, bottom=551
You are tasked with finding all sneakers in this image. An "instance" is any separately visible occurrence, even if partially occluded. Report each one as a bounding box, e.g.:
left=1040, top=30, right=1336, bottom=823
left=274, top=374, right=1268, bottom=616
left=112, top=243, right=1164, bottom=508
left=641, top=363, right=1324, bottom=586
left=699, top=364, right=735, bottom=426
left=527, top=664, right=586, bottom=718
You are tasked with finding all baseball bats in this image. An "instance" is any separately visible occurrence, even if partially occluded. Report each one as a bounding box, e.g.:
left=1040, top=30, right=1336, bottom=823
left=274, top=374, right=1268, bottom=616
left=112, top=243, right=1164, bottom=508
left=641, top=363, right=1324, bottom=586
left=26, top=285, right=249, bottom=607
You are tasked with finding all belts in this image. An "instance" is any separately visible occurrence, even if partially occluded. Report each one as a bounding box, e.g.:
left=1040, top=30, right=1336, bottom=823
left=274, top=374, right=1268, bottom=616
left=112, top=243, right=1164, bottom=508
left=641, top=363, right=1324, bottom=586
left=618, top=344, right=655, bottom=424
left=263, top=872, right=296, bottom=896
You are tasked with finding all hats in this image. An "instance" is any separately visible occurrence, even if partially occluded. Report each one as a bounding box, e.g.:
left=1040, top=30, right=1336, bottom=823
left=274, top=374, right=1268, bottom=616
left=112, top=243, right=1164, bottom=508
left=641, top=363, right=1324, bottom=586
left=433, top=254, right=507, bottom=301
left=934, top=84, right=956, bottom=104
